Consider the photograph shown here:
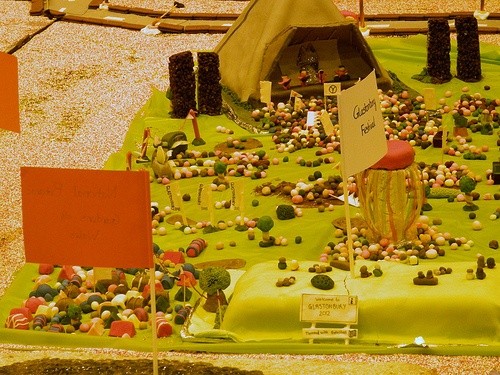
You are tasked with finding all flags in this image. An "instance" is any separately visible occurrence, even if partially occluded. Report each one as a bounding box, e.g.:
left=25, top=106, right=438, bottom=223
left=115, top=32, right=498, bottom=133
left=339, top=66, right=388, bottom=179
left=323, top=82, right=343, bottom=97
left=1, top=50, right=21, bottom=136
left=19, top=167, right=159, bottom=274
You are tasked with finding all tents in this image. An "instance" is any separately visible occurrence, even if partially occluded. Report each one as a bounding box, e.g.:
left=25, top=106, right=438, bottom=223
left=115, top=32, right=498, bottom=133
left=210, top=0, right=400, bottom=105
